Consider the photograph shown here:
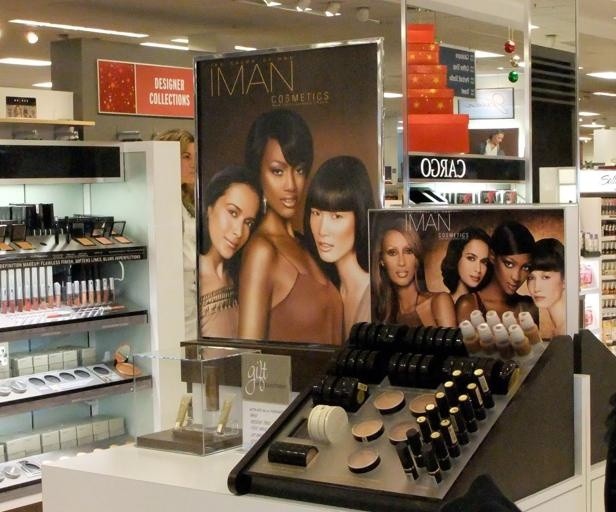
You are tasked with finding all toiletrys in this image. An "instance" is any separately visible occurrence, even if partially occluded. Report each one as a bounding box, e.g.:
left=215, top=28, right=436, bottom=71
left=172, top=392, right=195, bottom=432
left=216, top=398, right=236, bottom=436
left=579, top=195, right=616, bottom=349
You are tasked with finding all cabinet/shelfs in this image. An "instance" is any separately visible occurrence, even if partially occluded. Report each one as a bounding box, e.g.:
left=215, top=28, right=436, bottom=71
left=581, top=192, right=616, bottom=347
left=0, top=216, right=154, bottom=512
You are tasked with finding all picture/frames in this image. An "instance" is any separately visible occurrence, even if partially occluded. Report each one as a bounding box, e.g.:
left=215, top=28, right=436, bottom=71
left=458, top=86, right=514, bottom=120
left=367, top=204, right=569, bottom=345
left=193, top=37, right=386, bottom=345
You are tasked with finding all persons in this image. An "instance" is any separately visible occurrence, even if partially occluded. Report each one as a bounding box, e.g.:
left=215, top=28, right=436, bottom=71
left=371, top=218, right=458, bottom=329
left=198, top=162, right=264, bottom=337
left=524, top=235, right=568, bottom=334
left=150, top=126, right=198, bottom=341
left=236, top=107, right=342, bottom=341
left=302, top=152, right=378, bottom=341
left=454, top=220, right=540, bottom=333
left=476, top=130, right=511, bottom=155
left=436, top=224, right=493, bottom=307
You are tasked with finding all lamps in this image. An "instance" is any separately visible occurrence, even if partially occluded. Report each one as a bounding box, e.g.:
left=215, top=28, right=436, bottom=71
left=255, top=1, right=341, bottom=17
left=25, top=30, right=38, bottom=44
